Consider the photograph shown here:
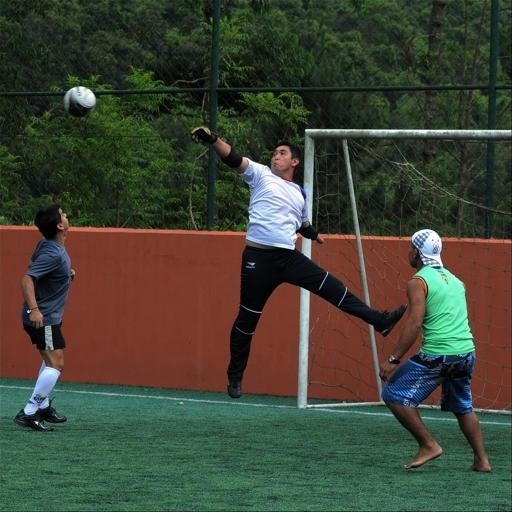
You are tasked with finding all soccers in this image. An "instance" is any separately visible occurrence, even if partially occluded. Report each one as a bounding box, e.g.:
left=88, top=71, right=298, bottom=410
left=63, top=87, right=95, bottom=117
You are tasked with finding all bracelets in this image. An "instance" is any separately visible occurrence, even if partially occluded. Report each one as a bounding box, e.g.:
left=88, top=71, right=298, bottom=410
left=27, top=306, right=38, bottom=314
left=222, top=147, right=243, bottom=169
left=301, top=225, right=318, bottom=241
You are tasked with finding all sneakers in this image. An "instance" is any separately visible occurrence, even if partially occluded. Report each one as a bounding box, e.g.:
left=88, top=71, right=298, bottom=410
left=227, top=376, right=241, bottom=398
left=374, top=303, right=408, bottom=337
left=13, top=409, right=53, bottom=431
left=36, top=407, right=66, bottom=422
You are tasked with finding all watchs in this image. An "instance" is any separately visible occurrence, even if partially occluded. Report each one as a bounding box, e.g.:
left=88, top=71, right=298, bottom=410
left=387, top=355, right=400, bottom=365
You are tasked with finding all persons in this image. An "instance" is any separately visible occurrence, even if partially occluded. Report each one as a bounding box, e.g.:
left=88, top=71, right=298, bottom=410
left=13, top=204, right=75, bottom=431
left=380, top=229, right=490, bottom=473
left=193, top=127, right=408, bottom=399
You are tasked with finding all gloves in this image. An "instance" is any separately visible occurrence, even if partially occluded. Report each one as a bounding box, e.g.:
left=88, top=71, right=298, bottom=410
left=191, top=126, right=217, bottom=145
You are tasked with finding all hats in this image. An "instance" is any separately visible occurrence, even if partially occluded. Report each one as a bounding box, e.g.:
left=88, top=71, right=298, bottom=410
left=411, top=228, right=443, bottom=268
